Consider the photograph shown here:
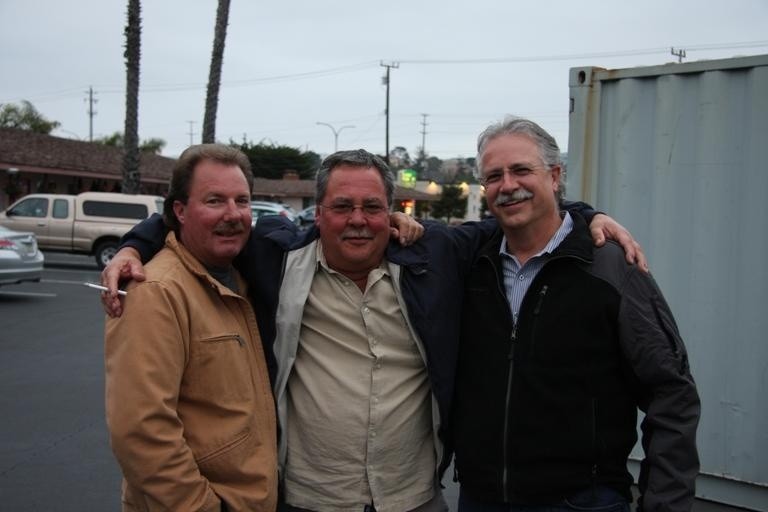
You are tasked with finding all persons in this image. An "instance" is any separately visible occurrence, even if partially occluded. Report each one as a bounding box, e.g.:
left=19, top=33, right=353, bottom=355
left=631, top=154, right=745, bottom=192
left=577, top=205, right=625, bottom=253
left=420, top=118, right=700, bottom=511
left=103, top=142, right=425, bottom=511
left=102, top=150, right=650, bottom=511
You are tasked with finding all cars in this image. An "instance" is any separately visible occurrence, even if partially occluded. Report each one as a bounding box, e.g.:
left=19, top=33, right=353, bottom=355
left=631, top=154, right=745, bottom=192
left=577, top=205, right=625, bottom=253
left=0, top=226, right=44, bottom=286
left=250, top=200, right=299, bottom=228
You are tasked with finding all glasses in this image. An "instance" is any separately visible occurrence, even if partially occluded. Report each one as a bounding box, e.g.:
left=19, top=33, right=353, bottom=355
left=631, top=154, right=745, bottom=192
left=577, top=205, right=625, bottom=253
left=319, top=202, right=391, bottom=217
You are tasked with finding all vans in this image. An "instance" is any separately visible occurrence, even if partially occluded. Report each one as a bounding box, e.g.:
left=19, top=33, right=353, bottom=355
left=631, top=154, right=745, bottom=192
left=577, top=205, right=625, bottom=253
left=0, top=190, right=165, bottom=270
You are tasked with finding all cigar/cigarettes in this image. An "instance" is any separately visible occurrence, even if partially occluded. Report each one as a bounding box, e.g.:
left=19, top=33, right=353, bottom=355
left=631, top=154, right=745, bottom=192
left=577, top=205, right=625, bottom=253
left=84, top=282, right=127, bottom=298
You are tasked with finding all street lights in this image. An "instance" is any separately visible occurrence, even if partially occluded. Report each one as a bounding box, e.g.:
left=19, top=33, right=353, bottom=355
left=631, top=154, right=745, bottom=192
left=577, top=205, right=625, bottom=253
left=426, top=181, right=470, bottom=227
left=316, top=121, right=354, bottom=151
